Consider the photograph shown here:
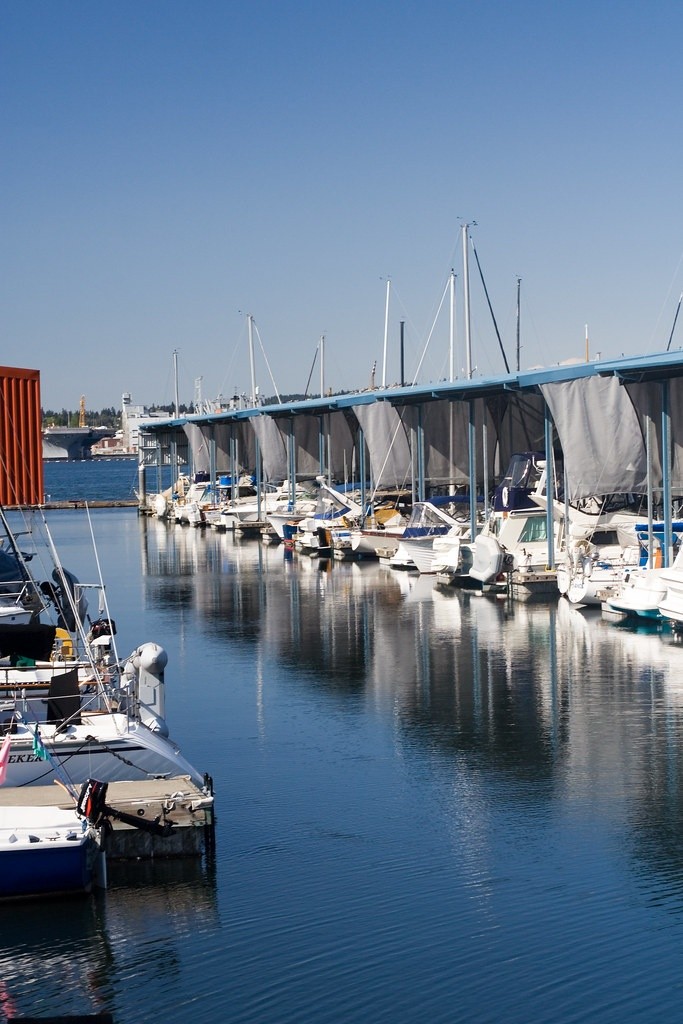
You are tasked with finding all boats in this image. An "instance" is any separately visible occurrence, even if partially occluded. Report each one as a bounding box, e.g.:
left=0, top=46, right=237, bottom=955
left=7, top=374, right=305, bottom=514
left=0, top=389, right=218, bottom=905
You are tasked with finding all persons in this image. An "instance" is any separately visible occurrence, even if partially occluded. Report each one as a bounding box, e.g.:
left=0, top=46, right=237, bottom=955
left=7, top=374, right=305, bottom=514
left=172, top=491, right=181, bottom=501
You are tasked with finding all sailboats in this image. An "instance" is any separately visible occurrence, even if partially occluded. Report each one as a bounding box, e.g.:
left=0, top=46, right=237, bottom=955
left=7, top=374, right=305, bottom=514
left=128, top=214, right=683, bottom=643
